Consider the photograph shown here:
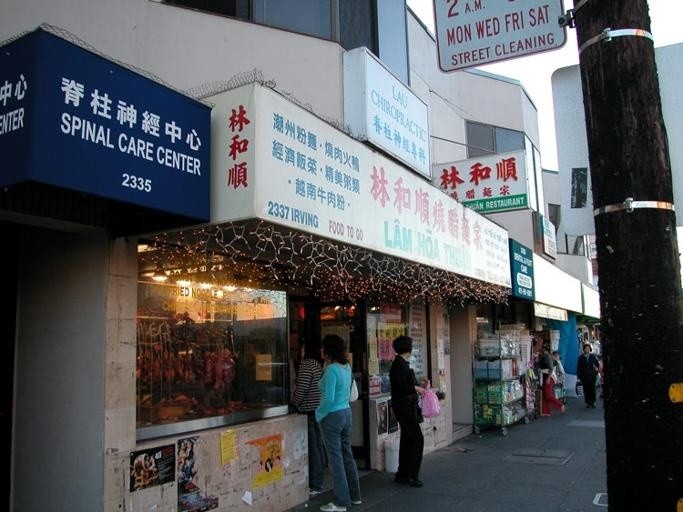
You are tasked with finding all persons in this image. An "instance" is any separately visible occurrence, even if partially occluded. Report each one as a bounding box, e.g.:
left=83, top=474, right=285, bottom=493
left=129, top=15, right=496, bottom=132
left=576, top=344, right=604, bottom=409
left=289, top=333, right=362, bottom=512
left=129, top=453, right=158, bottom=490
left=390, top=335, right=431, bottom=487
left=178, top=439, right=193, bottom=464
left=533, top=346, right=567, bottom=416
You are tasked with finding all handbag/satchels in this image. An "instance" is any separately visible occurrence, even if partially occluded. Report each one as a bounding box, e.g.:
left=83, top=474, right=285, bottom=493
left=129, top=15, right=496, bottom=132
left=422, top=389, right=440, bottom=417
left=349, top=380, right=358, bottom=402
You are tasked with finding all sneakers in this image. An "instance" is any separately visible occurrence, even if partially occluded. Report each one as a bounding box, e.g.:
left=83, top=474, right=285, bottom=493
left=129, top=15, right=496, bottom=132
left=394, top=472, right=423, bottom=488
left=560, top=404, right=565, bottom=415
left=309, top=488, right=322, bottom=494
left=541, top=412, right=552, bottom=416
left=351, top=499, right=361, bottom=505
left=586, top=402, right=596, bottom=409
left=320, top=503, right=347, bottom=512
